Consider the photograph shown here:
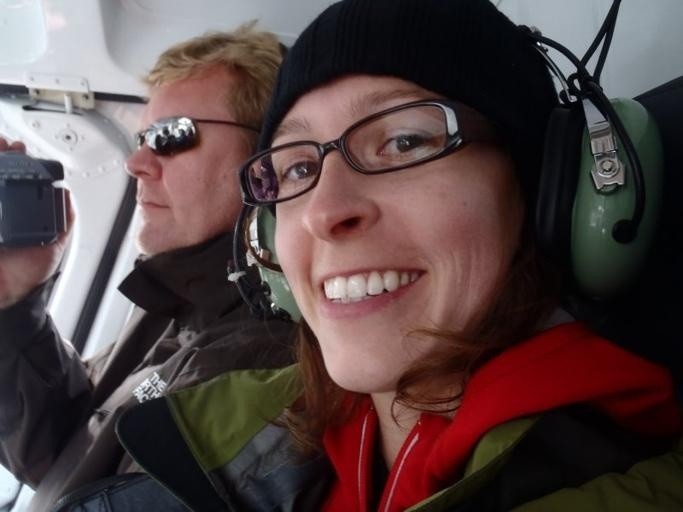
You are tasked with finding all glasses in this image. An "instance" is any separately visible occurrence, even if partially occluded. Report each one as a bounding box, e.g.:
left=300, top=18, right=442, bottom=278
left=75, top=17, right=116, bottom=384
left=134, top=116, right=261, bottom=155
left=238, top=98, right=493, bottom=207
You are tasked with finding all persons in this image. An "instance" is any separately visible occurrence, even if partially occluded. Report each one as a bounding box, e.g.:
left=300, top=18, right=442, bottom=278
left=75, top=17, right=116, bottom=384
left=238, top=0, right=683, bottom=512
left=0, top=29, right=304, bottom=511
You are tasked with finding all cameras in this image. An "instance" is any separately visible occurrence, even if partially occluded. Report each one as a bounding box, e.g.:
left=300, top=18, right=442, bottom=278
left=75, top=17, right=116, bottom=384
left=0, top=150, right=71, bottom=248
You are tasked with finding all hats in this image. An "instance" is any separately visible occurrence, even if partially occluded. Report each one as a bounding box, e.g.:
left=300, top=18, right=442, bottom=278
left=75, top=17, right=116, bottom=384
left=257, top=0, right=562, bottom=195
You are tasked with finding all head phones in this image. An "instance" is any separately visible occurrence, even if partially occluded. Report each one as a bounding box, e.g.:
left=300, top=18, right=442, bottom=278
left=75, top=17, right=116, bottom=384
left=225, top=24, right=665, bottom=328
